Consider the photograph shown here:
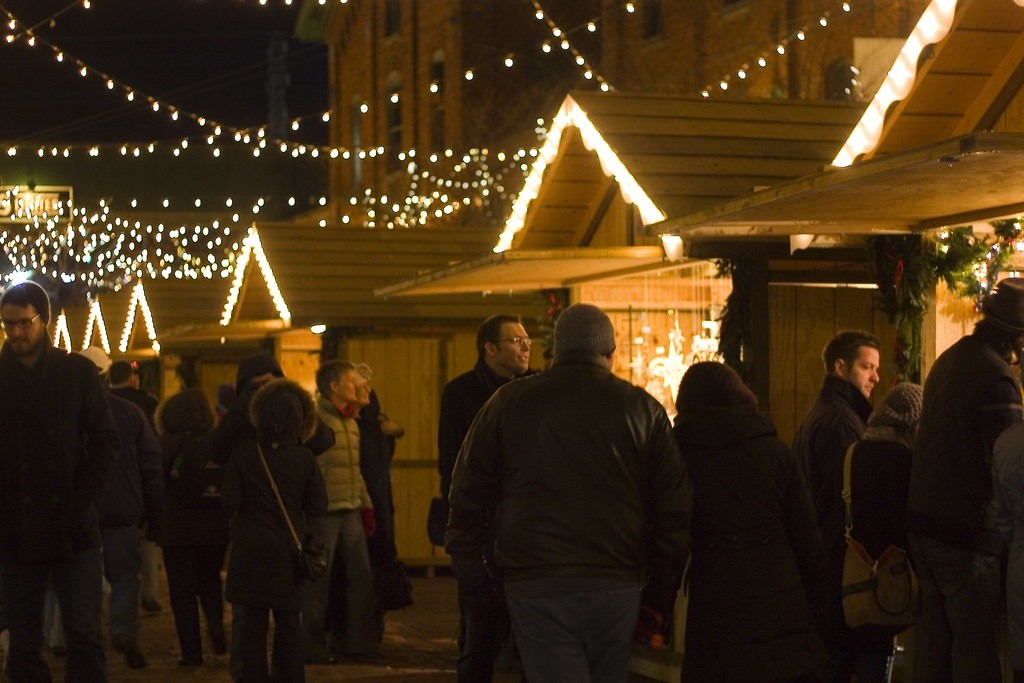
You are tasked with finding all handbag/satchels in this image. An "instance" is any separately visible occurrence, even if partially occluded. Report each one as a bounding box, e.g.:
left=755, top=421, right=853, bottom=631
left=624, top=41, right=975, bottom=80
left=425, top=495, right=450, bottom=550
left=672, top=587, right=690, bottom=654
left=294, top=532, right=332, bottom=587
left=385, top=557, right=414, bottom=610
left=842, top=537, right=917, bottom=632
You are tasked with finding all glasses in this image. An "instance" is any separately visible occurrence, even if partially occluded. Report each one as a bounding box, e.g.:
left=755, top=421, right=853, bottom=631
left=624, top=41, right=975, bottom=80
left=492, top=336, right=534, bottom=346
left=1, top=313, right=39, bottom=331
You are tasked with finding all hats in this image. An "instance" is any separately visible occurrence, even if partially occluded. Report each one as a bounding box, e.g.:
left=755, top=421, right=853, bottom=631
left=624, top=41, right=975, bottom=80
left=82, top=347, right=113, bottom=375
left=556, top=303, right=617, bottom=354
left=982, top=277, right=1024, bottom=334
left=872, top=382, right=923, bottom=430
left=675, top=362, right=760, bottom=412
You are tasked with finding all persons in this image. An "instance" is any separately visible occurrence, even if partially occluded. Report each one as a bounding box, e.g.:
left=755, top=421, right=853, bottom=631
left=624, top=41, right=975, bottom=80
left=105, top=359, right=336, bottom=665
left=437, top=315, right=540, bottom=683
left=675, top=362, right=830, bottom=683
left=908, top=278, right=1024, bottom=683
left=0, top=283, right=120, bottom=683
left=304, top=361, right=405, bottom=663
left=444, top=306, right=693, bottom=683
left=822, top=383, right=923, bottom=683
left=993, top=421, right=1024, bottom=683
left=51, top=345, right=162, bottom=669
left=794, top=328, right=880, bottom=578
left=220, top=378, right=328, bottom=683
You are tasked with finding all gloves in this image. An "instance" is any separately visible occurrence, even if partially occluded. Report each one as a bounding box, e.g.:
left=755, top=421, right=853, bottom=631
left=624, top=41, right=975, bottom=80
left=364, top=507, right=376, bottom=538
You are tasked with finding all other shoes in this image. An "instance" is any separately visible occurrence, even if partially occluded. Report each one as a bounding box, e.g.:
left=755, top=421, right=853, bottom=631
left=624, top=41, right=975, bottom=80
left=344, top=651, right=387, bottom=664
left=139, top=597, right=161, bottom=615
left=213, top=641, right=226, bottom=657
left=117, top=639, right=145, bottom=668
left=306, top=653, right=338, bottom=666
left=178, top=658, right=202, bottom=666
